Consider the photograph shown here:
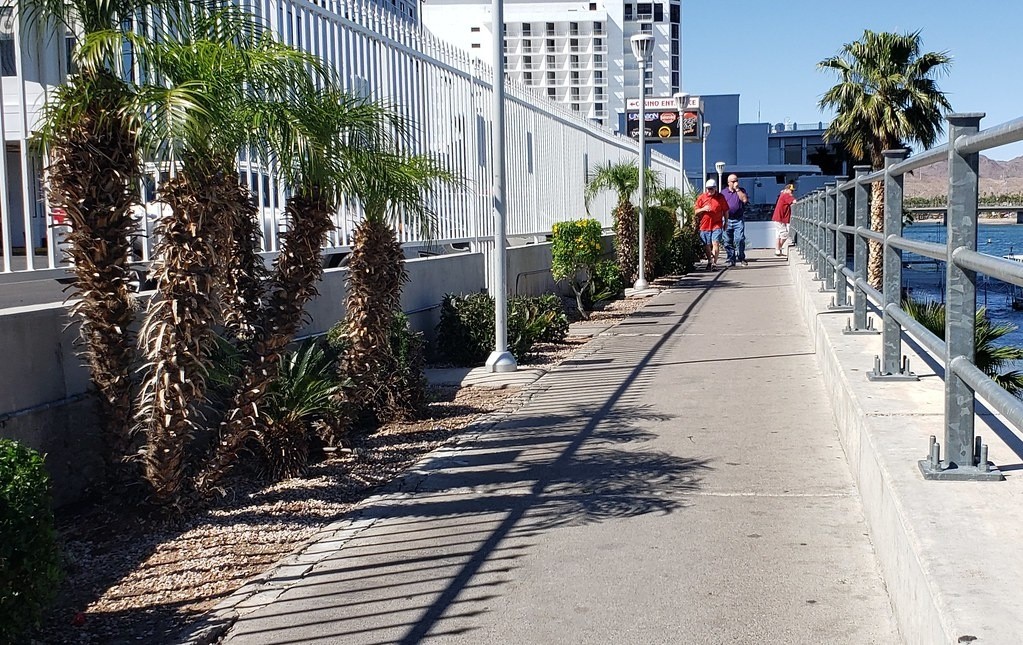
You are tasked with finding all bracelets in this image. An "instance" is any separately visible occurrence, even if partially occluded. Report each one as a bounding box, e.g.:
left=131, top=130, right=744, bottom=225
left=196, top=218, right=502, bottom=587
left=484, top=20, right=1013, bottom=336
left=736, top=189, right=740, bottom=192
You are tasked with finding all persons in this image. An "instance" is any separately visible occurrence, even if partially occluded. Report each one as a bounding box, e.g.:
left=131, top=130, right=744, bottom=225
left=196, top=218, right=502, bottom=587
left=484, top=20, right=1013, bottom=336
left=720, top=174, right=749, bottom=267
left=694, top=178, right=729, bottom=272
left=772, top=184, right=797, bottom=256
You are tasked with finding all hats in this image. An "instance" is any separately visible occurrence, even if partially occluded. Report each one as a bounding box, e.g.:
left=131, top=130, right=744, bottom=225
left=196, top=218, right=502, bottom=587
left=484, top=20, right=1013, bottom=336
left=787, top=184, right=797, bottom=191
left=706, top=179, right=716, bottom=189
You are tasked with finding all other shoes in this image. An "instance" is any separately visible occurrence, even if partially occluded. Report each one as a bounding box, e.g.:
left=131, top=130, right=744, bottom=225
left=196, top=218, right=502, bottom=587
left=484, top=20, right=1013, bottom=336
left=706, top=261, right=712, bottom=271
left=741, top=259, right=748, bottom=266
left=775, top=250, right=785, bottom=257
left=712, top=263, right=717, bottom=272
left=727, top=261, right=735, bottom=267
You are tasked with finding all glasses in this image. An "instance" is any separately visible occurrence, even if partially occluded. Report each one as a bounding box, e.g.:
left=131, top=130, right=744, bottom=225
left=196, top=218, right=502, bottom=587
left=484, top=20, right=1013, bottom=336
left=729, top=180, right=738, bottom=183
